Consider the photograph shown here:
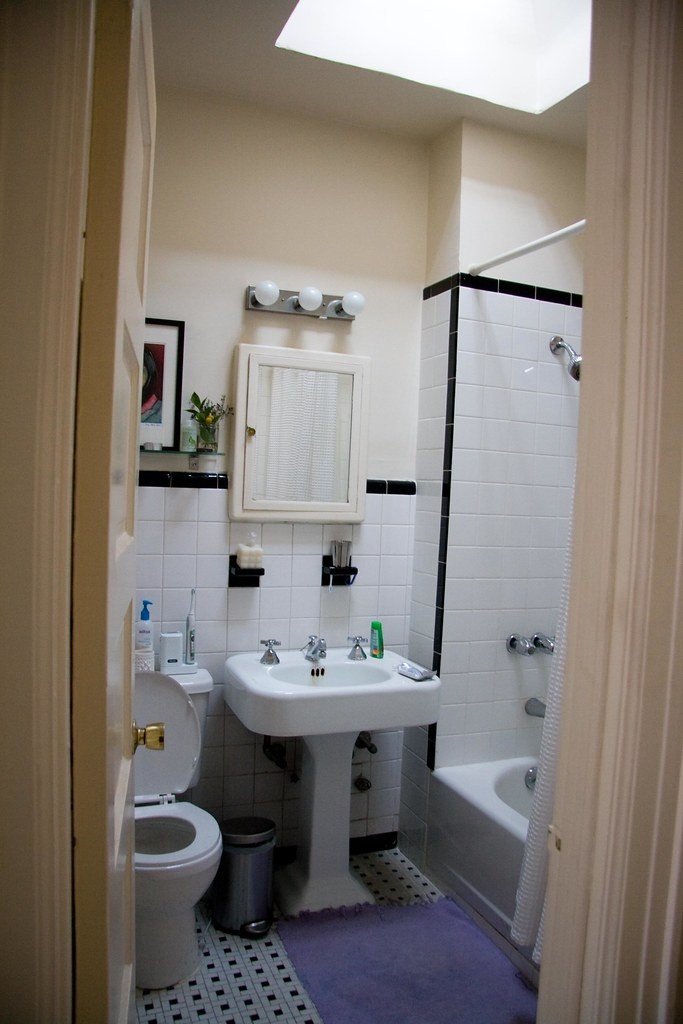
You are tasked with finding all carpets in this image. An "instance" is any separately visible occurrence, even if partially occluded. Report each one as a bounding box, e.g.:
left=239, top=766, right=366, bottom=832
left=279, top=897, right=538, bottom=1024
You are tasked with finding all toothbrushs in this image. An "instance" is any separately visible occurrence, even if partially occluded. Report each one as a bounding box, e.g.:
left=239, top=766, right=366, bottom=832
left=328, top=567, right=335, bottom=591
left=186, top=589, right=196, bottom=664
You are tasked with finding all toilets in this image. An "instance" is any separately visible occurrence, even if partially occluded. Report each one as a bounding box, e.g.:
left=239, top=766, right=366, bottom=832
left=132, top=668, right=224, bottom=990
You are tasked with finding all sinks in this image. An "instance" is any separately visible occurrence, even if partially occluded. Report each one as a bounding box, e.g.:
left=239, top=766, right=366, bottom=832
left=224, top=645, right=442, bottom=737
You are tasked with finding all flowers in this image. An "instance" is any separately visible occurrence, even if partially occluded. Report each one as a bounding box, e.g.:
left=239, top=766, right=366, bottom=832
left=186, top=392, right=234, bottom=449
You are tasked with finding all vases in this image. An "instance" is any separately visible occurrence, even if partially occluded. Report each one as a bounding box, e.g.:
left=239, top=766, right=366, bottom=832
left=196, top=423, right=220, bottom=453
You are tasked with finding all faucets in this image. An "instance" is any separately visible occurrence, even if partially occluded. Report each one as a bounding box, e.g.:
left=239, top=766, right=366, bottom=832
left=303, top=635, right=327, bottom=662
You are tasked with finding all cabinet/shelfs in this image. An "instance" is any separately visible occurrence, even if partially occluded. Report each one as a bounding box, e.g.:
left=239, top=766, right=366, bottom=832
left=229, top=342, right=366, bottom=524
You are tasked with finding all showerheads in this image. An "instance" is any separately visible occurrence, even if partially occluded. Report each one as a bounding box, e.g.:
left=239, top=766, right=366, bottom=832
left=548, top=335, right=581, bottom=381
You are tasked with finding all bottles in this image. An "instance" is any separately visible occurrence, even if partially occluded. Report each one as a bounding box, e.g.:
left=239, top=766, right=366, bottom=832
left=370, top=621, right=384, bottom=658
left=180, top=419, right=197, bottom=452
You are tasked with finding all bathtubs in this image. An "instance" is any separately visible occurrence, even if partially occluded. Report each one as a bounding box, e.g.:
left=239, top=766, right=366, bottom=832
left=429, top=754, right=544, bottom=973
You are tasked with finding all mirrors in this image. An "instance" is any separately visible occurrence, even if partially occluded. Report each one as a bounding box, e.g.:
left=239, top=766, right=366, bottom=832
left=252, top=365, right=354, bottom=503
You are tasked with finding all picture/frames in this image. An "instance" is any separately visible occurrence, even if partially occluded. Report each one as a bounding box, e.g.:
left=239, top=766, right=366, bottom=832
left=140, top=318, right=185, bottom=452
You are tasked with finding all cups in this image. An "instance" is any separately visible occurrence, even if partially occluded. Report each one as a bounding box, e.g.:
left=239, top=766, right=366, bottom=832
left=135, top=650, right=154, bottom=672
left=331, top=539, right=353, bottom=568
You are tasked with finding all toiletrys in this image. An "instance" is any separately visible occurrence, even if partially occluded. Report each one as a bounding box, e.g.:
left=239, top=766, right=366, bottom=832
left=370, top=621, right=385, bottom=659
left=136, top=600, right=153, bottom=650
left=397, top=661, right=438, bottom=681
